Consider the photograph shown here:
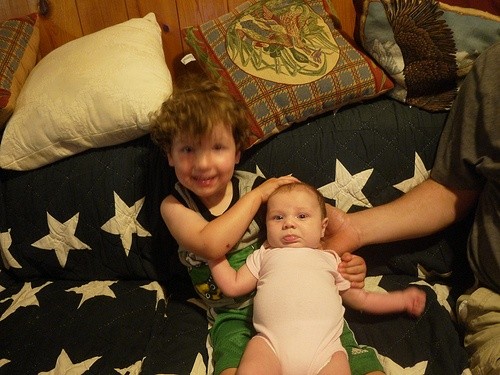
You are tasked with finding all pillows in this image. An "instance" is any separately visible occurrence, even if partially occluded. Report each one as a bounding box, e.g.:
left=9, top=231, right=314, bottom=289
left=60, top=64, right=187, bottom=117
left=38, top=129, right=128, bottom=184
left=359, top=0, right=500, bottom=111
left=181, top=0, right=394, bottom=150
left=0, top=11, right=40, bottom=127
left=0, top=12, right=174, bottom=171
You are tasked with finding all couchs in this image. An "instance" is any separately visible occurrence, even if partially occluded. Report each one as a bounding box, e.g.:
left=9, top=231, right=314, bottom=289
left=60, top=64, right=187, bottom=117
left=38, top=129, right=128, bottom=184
left=0, top=91, right=477, bottom=375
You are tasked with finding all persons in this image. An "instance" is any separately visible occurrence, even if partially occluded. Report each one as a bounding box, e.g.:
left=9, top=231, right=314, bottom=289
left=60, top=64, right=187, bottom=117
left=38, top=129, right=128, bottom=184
left=207, top=180, right=431, bottom=375
left=262, top=41, right=500, bottom=375
left=155, top=82, right=387, bottom=375
